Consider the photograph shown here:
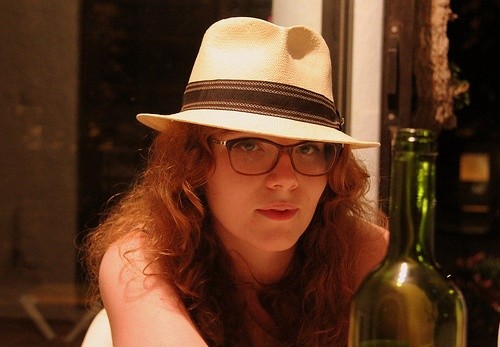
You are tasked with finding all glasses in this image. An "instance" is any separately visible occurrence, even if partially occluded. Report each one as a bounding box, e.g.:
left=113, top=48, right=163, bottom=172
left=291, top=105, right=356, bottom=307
left=208, top=135, right=339, bottom=176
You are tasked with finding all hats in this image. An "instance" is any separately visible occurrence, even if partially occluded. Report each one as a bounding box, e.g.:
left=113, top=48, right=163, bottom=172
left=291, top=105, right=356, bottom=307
left=134, top=17, right=381, bottom=152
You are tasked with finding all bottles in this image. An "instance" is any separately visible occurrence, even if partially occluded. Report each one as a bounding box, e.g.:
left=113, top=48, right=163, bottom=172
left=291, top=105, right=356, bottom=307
left=346, top=127, right=468, bottom=347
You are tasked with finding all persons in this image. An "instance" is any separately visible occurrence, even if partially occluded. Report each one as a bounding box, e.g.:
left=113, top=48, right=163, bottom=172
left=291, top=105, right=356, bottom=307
left=83, top=17, right=389, bottom=347
